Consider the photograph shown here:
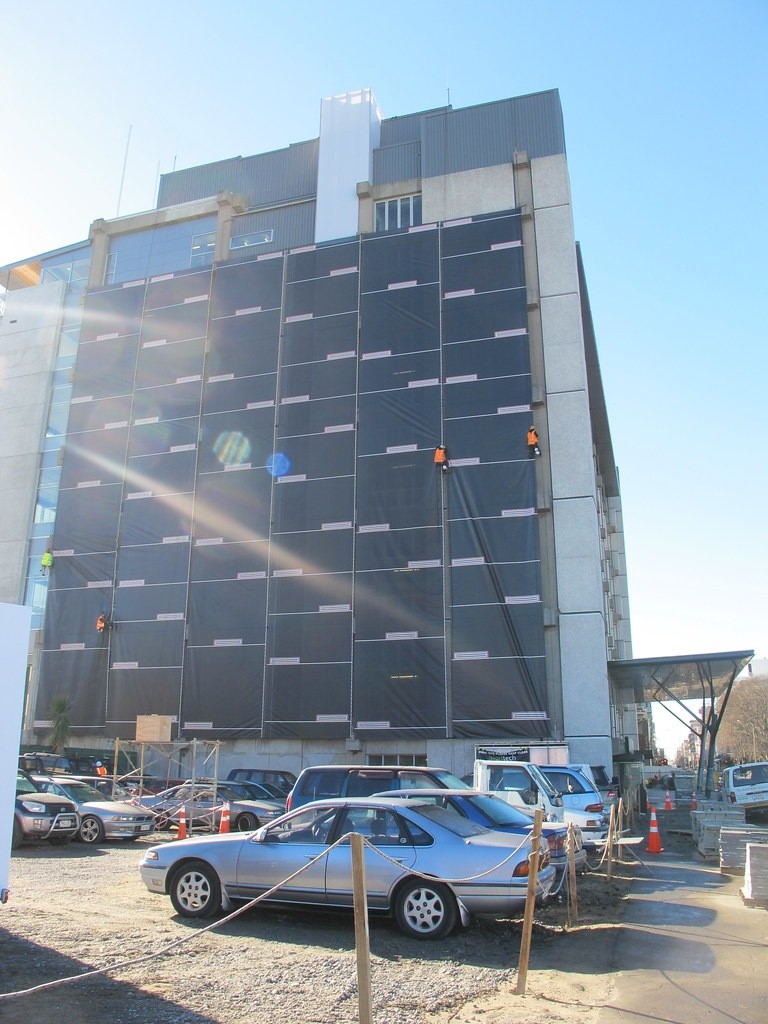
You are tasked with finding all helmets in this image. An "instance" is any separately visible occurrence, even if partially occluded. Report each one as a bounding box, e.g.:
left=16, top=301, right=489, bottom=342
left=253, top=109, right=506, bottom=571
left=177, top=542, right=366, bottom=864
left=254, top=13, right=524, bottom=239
left=96, top=761, right=102, bottom=766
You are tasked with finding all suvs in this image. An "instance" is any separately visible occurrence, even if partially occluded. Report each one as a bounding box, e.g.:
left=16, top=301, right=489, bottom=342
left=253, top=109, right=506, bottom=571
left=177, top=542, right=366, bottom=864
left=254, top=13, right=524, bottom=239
left=286, top=764, right=557, bottom=832
left=12, top=768, right=81, bottom=848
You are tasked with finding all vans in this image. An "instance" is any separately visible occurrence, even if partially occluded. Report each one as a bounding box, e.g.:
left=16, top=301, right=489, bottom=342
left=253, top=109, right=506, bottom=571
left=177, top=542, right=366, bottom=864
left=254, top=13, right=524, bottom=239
left=717, top=762, right=768, bottom=819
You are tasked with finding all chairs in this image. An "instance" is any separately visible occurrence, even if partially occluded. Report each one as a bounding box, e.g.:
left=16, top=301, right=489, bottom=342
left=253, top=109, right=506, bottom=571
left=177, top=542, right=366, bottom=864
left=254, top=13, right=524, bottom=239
left=366, top=819, right=388, bottom=845
left=338, top=819, right=352, bottom=846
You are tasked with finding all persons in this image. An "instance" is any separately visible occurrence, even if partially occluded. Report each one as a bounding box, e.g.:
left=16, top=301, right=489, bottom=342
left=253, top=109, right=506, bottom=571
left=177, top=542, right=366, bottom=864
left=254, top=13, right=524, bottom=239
left=41, top=549, right=52, bottom=568
left=434, top=446, right=449, bottom=470
left=527, top=426, right=539, bottom=460
left=96, top=761, right=107, bottom=775
left=96, top=612, right=107, bottom=630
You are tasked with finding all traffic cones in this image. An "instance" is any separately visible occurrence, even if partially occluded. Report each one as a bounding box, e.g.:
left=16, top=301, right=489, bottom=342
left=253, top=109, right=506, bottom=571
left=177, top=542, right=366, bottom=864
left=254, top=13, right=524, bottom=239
left=665, top=789, right=672, bottom=810
left=219, top=801, right=231, bottom=834
left=646, top=793, right=650, bottom=809
left=691, top=791, right=698, bottom=811
left=177, top=803, right=186, bottom=840
left=645, top=807, right=664, bottom=852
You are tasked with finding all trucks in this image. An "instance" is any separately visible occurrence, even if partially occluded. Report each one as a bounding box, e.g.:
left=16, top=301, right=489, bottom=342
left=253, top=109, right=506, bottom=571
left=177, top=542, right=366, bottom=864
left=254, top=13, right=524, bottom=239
left=466, top=759, right=564, bottom=823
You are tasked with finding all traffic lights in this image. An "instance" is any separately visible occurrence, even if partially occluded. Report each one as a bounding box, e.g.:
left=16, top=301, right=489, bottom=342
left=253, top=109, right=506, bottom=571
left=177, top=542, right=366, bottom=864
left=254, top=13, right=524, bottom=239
left=658, top=759, right=666, bottom=764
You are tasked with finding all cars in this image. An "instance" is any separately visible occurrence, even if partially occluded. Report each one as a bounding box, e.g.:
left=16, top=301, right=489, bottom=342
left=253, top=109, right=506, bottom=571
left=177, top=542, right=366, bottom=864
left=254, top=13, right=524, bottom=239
left=183, top=769, right=297, bottom=810
left=54, top=775, right=132, bottom=802
left=140, top=796, right=555, bottom=940
left=17, top=774, right=156, bottom=844
left=557, top=763, right=620, bottom=814
left=135, top=784, right=284, bottom=833
left=369, top=789, right=586, bottom=874
left=460, top=765, right=604, bottom=818
left=564, top=808, right=609, bottom=846
